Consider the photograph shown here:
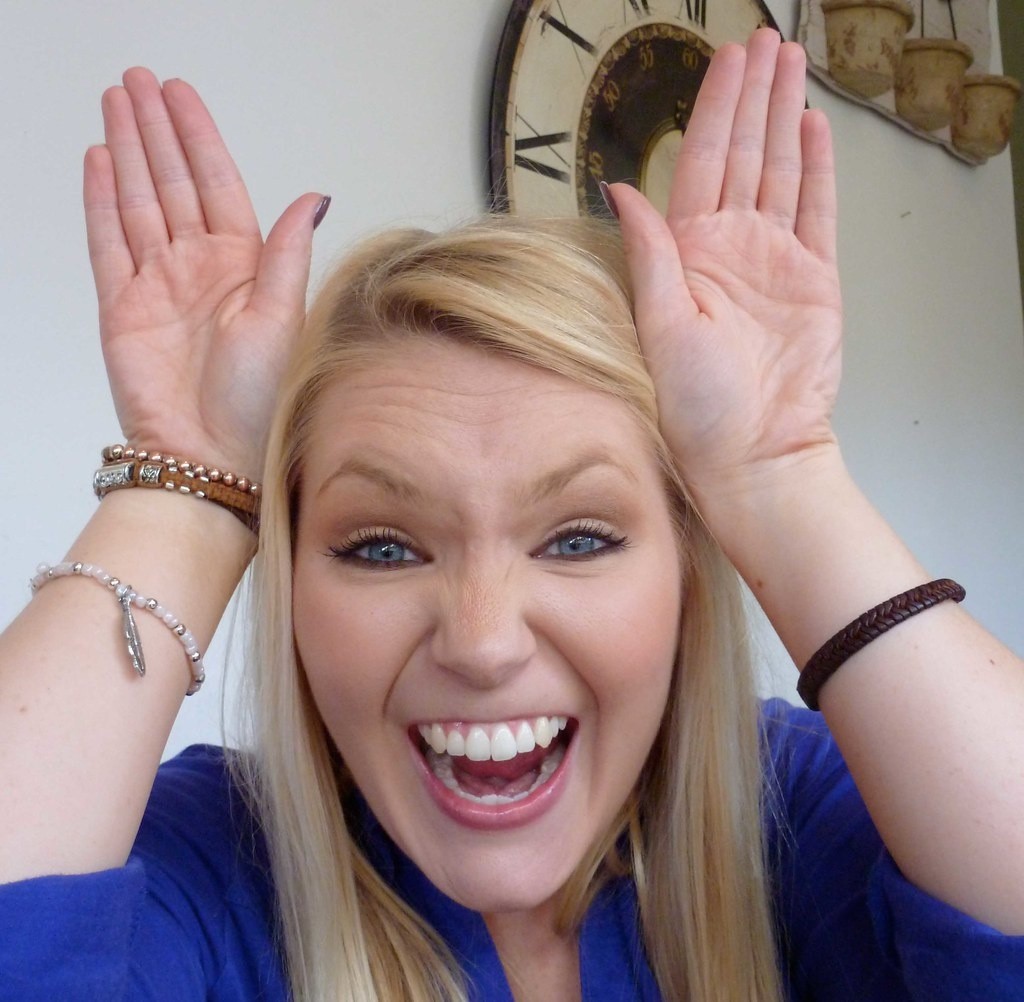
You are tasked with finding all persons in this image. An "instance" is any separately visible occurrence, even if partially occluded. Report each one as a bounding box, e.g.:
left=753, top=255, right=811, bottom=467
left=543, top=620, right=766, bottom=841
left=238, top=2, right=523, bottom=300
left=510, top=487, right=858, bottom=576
left=0, top=28, right=1023, bottom=1002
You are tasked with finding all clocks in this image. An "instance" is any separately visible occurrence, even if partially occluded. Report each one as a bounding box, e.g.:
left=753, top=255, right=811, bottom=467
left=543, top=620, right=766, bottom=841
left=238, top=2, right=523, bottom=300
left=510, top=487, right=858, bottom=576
left=489, top=1, right=813, bottom=223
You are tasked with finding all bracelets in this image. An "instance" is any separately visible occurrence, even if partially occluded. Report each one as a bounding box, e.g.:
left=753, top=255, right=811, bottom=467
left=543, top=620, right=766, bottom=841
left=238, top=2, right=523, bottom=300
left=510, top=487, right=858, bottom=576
left=29, top=562, right=205, bottom=696
left=93, top=444, right=262, bottom=536
left=796, top=579, right=966, bottom=711
